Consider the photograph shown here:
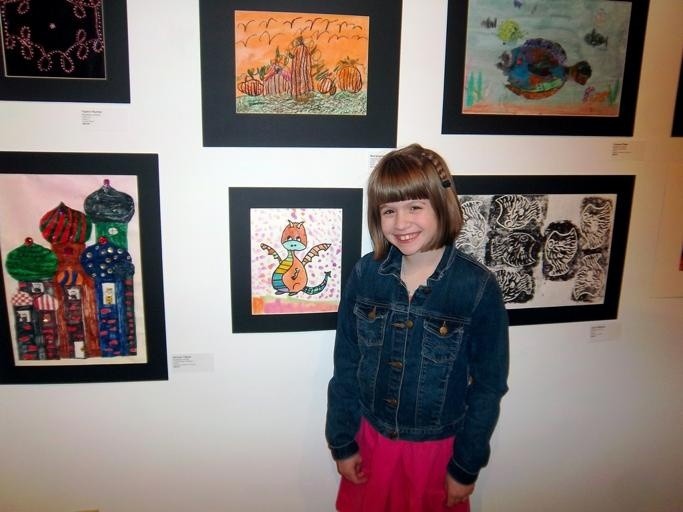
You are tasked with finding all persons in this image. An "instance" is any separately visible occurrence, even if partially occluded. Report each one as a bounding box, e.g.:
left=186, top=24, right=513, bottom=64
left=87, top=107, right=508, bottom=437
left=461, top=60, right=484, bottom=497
left=325, top=143, right=510, bottom=512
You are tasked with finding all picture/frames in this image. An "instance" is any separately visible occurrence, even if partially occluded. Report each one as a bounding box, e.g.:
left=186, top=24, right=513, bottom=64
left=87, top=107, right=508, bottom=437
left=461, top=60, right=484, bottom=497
left=0, top=0, right=130, bottom=105
left=199, top=0, right=402, bottom=148
left=228, top=186, right=363, bottom=334
left=0, top=152, right=170, bottom=383
left=441, top=0, right=650, bottom=136
left=451, top=173, right=636, bottom=326
